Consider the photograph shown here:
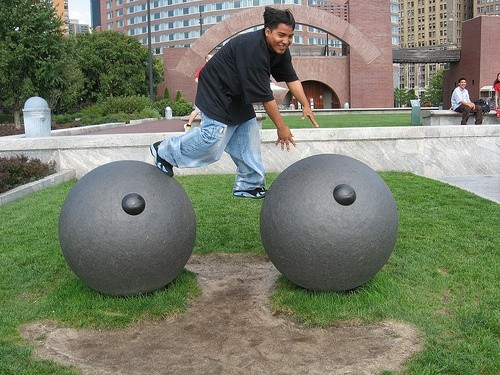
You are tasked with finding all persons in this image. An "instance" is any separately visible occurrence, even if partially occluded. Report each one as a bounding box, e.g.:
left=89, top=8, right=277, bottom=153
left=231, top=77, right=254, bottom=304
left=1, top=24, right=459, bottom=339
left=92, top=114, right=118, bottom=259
left=450, top=78, right=483, bottom=125
left=492, top=73, right=500, bottom=110
left=183, top=54, right=213, bottom=133
left=149, top=7, right=319, bottom=199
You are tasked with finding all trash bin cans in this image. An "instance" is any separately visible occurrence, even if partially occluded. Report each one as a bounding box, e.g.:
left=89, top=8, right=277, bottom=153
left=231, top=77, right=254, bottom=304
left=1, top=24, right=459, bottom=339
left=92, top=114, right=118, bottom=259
left=22, top=96, right=51, bottom=137
left=165, top=107, right=172, bottom=120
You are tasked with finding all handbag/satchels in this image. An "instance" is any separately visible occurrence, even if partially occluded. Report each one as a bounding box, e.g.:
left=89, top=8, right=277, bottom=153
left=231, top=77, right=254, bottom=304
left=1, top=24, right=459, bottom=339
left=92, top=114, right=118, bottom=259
left=474, top=98, right=490, bottom=115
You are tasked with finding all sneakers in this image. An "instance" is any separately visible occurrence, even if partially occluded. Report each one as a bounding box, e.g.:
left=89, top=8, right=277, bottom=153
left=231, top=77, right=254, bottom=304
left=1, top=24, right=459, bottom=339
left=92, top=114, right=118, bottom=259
left=150, top=140, right=175, bottom=177
left=232, top=187, right=267, bottom=199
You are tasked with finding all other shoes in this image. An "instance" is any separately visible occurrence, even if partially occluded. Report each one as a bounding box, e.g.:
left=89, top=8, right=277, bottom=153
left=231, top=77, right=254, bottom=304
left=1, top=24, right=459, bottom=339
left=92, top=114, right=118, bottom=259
left=183, top=123, right=192, bottom=133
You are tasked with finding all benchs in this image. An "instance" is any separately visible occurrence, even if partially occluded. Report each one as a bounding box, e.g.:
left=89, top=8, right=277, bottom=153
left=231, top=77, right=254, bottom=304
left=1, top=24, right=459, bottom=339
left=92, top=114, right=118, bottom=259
left=181, top=113, right=266, bottom=129
left=422, top=109, right=500, bottom=126
